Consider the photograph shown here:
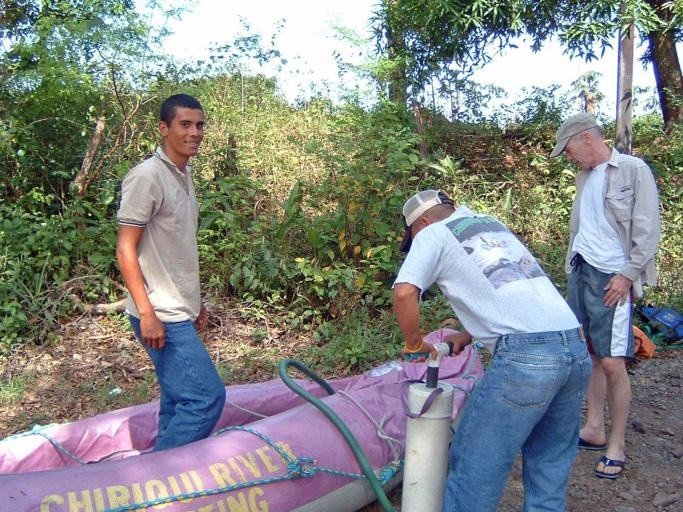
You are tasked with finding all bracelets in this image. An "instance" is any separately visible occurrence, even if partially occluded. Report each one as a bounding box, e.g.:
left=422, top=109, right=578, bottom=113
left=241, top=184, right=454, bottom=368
left=405, top=340, right=423, bottom=352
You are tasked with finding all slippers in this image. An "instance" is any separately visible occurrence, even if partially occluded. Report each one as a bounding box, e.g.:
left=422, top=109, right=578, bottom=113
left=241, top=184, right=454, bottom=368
left=578, top=436, right=626, bottom=479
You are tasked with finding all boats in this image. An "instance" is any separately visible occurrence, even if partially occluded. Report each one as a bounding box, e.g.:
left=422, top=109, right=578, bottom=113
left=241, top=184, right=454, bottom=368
left=1, top=329, right=484, bottom=507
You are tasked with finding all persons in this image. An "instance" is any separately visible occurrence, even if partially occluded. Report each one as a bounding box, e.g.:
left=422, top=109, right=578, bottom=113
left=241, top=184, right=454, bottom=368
left=116, top=94, right=226, bottom=451
left=549, top=114, right=658, bottom=478
left=391, top=189, right=592, bottom=512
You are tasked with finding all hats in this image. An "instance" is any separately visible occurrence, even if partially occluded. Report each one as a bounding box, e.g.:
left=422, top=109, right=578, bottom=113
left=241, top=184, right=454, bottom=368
left=549, top=113, right=598, bottom=158
left=399, top=189, right=454, bottom=252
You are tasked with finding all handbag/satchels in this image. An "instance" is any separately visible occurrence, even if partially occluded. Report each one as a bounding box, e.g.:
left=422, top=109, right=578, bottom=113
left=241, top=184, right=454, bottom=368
left=637, top=306, right=682, bottom=340
left=632, top=325, right=657, bottom=359
